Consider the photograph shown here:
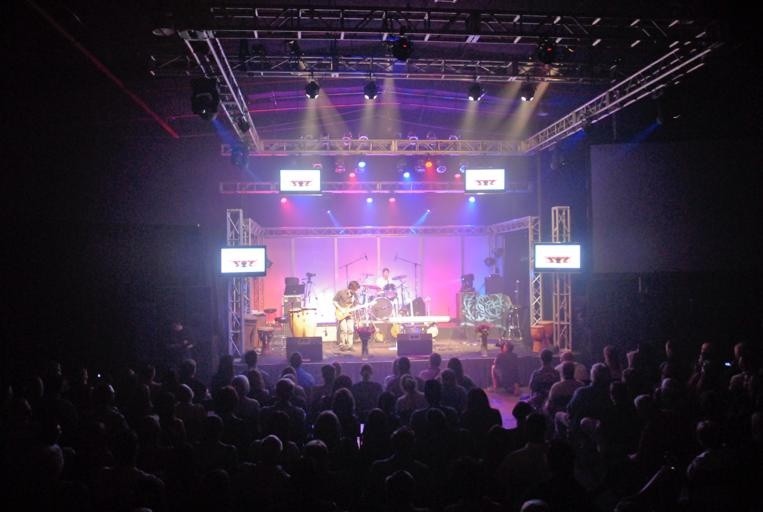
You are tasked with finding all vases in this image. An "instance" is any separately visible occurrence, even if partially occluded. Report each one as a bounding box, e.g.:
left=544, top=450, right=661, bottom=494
left=480, top=336, right=488, bottom=357
left=361, top=344, right=368, bottom=360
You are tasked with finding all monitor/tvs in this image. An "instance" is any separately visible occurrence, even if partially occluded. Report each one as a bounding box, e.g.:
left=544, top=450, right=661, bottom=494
left=219, top=246, right=267, bottom=276
left=464, top=167, right=506, bottom=192
left=534, top=242, right=582, bottom=272
left=279, top=168, right=321, bottom=194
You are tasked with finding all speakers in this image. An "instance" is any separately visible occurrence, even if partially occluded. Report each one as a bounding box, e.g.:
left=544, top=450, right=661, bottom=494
left=286, top=336, right=322, bottom=363
left=396, top=334, right=432, bottom=355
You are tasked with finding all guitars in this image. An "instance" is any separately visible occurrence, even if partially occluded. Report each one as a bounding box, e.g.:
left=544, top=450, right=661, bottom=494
left=335, top=302, right=376, bottom=320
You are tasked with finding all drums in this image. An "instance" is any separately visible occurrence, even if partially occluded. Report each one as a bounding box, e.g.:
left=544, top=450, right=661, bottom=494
left=288, top=307, right=316, bottom=337
left=243, top=314, right=274, bottom=356
left=362, top=285, right=396, bottom=318
left=530, top=320, right=553, bottom=353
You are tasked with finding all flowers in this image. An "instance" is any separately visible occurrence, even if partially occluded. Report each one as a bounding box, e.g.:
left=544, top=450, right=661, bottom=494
left=355, top=325, right=376, bottom=355
left=474, top=323, right=496, bottom=351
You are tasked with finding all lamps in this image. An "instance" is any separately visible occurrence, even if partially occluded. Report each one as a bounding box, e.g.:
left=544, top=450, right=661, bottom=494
left=537, top=41, right=558, bottom=64
left=364, top=81, right=380, bottom=101
left=391, top=36, right=414, bottom=63
left=520, top=85, right=535, bottom=102
left=304, top=80, right=320, bottom=100
left=468, top=83, right=486, bottom=102
left=190, top=73, right=220, bottom=120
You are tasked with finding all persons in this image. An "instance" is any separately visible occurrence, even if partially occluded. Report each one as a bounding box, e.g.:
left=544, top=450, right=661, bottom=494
left=331, top=280, right=366, bottom=351
left=375, top=266, right=394, bottom=289
left=0, top=321, right=763, bottom=509
